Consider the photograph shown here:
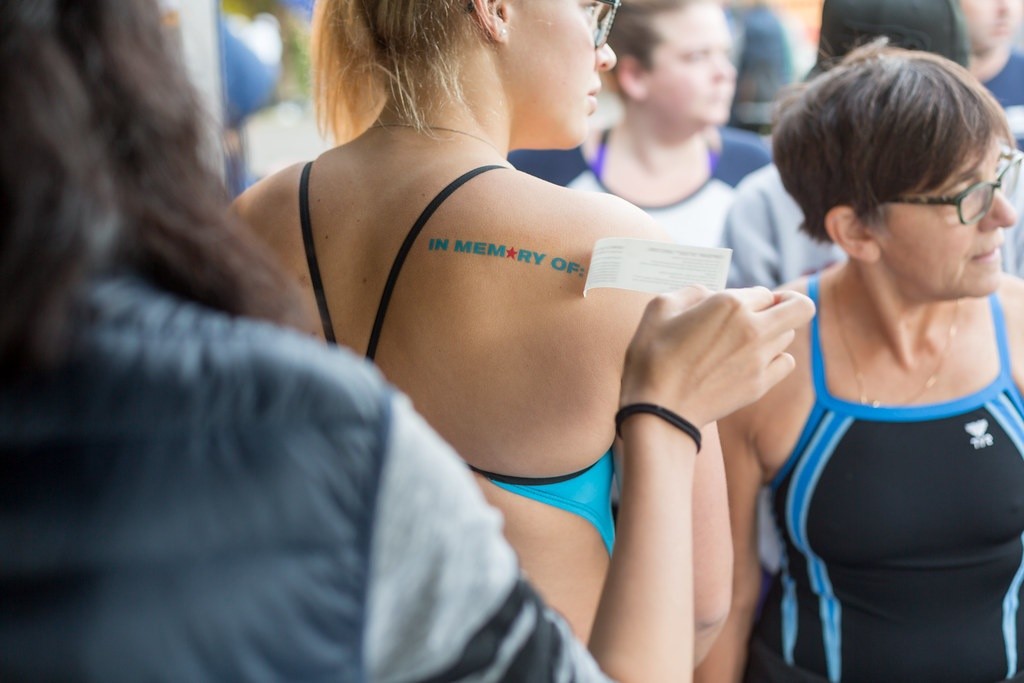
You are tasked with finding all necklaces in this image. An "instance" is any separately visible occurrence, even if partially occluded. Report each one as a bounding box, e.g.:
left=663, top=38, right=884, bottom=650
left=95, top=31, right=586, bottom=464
left=364, top=120, right=502, bottom=157
left=833, top=290, right=957, bottom=411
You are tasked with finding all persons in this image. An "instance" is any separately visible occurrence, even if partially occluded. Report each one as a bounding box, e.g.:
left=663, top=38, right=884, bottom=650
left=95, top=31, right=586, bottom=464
left=511, top=3, right=1020, bottom=683
left=1, top=3, right=820, bottom=681
left=208, top=0, right=740, bottom=681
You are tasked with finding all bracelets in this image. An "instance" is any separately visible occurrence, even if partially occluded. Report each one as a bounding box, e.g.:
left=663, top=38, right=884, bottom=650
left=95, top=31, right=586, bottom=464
left=616, top=403, right=704, bottom=458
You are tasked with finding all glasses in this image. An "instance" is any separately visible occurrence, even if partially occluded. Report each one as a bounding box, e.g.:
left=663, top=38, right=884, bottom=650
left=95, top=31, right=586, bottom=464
left=883, top=147, right=1023, bottom=224
left=596, top=0, right=623, bottom=49
